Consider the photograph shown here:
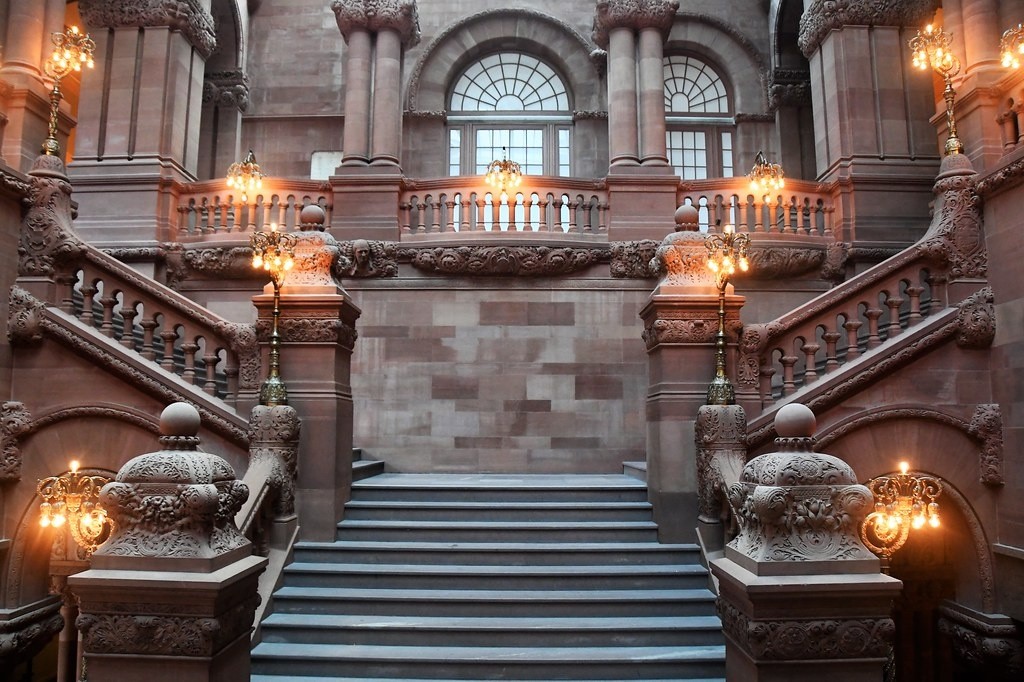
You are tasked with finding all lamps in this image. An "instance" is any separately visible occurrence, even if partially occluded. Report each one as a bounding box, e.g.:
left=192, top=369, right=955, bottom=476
left=226, top=150, right=267, bottom=201
left=704, top=224, right=753, bottom=405
left=749, top=150, right=784, bottom=189
left=36, top=459, right=116, bottom=682
left=907, top=24, right=965, bottom=155
left=40, top=25, right=96, bottom=156
left=484, top=159, right=522, bottom=189
left=249, top=223, right=301, bottom=407
left=861, top=461, right=943, bottom=578
left=999, top=23, right=1024, bottom=69
